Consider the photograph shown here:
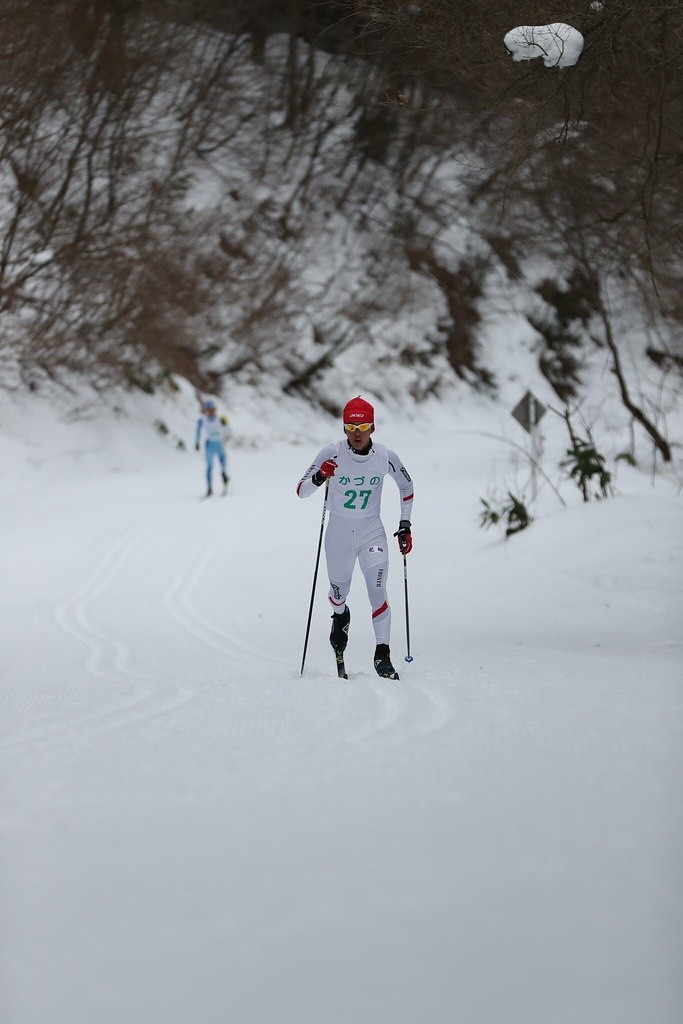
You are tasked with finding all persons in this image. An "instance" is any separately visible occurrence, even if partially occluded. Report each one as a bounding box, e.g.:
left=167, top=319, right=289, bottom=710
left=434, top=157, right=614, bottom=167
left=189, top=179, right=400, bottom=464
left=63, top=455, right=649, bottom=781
left=296, top=395, right=414, bottom=676
left=193, top=400, right=233, bottom=497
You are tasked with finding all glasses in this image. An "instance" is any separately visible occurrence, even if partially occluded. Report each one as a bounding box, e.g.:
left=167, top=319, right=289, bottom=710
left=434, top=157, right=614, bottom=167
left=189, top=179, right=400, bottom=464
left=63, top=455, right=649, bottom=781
left=344, top=422, right=373, bottom=433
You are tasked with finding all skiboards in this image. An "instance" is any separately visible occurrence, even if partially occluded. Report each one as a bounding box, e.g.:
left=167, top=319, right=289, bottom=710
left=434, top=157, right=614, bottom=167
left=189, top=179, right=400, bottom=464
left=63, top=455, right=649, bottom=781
left=335, top=651, right=399, bottom=680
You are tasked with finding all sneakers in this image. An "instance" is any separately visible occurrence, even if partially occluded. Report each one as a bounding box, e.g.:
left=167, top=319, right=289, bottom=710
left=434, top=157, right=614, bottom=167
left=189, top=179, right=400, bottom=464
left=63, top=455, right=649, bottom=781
left=330, top=604, right=350, bottom=652
left=373, top=643, right=395, bottom=678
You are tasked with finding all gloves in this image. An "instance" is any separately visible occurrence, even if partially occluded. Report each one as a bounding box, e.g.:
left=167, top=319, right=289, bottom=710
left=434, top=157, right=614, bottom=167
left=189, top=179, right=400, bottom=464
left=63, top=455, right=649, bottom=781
left=312, top=460, right=338, bottom=486
left=394, top=520, right=412, bottom=555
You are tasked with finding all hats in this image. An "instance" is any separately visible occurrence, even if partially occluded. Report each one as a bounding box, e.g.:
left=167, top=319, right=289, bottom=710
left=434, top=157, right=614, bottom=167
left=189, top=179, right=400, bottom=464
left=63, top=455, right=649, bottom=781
left=203, top=401, right=214, bottom=410
left=343, top=395, right=374, bottom=423
